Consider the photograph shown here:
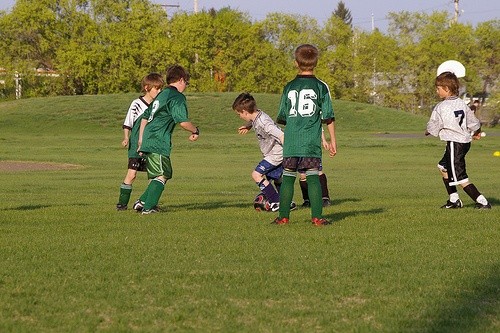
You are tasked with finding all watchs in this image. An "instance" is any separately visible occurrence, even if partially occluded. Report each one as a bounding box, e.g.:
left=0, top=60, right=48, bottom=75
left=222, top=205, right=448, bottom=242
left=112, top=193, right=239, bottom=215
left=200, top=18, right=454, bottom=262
left=192, top=129, right=199, bottom=135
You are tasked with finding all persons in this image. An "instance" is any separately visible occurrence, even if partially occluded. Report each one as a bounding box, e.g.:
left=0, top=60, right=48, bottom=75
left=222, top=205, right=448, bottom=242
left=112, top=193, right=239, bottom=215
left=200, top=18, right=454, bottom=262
left=425, top=72, right=492, bottom=209
left=232, top=93, right=297, bottom=212
left=117, top=73, right=165, bottom=211
left=470, top=100, right=480, bottom=113
left=133, top=66, right=199, bottom=213
left=271, top=44, right=337, bottom=225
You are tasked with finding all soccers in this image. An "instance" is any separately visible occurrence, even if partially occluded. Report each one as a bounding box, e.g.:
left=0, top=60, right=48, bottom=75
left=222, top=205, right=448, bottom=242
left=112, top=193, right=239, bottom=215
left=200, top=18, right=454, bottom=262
left=254, top=192, right=271, bottom=211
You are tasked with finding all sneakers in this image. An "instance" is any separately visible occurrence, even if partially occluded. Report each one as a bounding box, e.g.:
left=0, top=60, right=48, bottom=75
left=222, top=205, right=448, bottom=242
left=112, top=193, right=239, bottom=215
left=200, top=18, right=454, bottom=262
left=133, top=199, right=145, bottom=210
left=270, top=217, right=289, bottom=225
left=141, top=208, right=161, bottom=214
left=474, top=201, right=492, bottom=209
left=311, top=217, right=330, bottom=225
left=440, top=199, right=463, bottom=209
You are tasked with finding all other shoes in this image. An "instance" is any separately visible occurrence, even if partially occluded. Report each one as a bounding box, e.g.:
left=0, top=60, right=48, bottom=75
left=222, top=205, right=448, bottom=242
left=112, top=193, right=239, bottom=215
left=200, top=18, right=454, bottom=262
left=117, top=204, right=127, bottom=211
left=322, top=197, right=330, bottom=206
left=267, top=202, right=279, bottom=212
left=290, top=200, right=297, bottom=211
left=301, top=200, right=310, bottom=208
